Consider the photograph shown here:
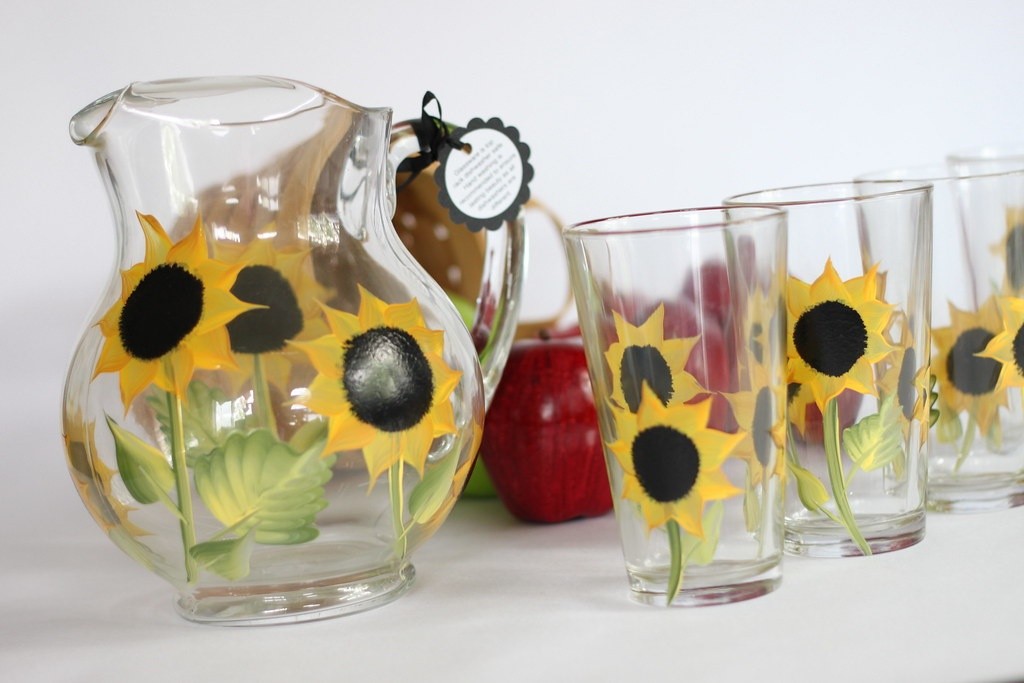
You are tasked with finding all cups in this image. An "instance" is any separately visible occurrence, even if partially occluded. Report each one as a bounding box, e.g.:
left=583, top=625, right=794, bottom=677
left=563, top=206, right=788, bottom=607
left=722, top=181, right=934, bottom=557
left=856, top=168, right=1024, bottom=512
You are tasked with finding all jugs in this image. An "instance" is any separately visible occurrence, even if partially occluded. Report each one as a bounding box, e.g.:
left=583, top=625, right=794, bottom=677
left=64, top=78, right=526, bottom=626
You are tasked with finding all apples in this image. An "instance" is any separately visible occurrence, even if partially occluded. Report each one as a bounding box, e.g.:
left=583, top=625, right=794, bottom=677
left=473, top=259, right=863, bottom=524
